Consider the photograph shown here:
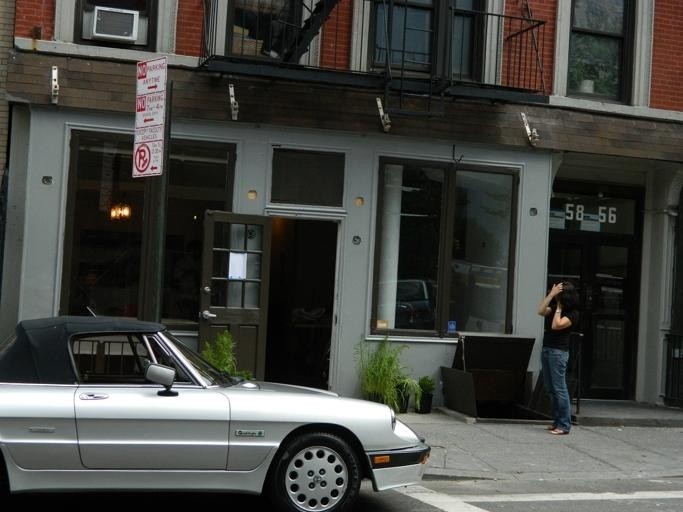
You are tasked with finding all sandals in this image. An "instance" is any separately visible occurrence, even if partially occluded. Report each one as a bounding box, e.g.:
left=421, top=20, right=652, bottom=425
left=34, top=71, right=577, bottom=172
left=550, top=428, right=569, bottom=435
left=544, top=424, right=555, bottom=431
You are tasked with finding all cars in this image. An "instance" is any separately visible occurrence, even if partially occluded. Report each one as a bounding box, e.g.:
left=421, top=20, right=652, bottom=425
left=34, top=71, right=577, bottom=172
left=1, top=306, right=436, bottom=511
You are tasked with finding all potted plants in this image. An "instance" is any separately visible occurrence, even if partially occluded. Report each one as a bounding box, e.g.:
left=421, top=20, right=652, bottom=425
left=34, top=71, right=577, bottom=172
left=352, top=332, right=436, bottom=413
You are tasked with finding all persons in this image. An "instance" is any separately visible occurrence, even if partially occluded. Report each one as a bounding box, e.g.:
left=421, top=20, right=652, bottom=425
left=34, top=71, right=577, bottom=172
left=538, top=279, right=577, bottom=436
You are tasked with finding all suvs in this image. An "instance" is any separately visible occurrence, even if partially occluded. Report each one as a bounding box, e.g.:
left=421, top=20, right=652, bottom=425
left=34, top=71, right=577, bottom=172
left=370, top=276, right=457, bottom=333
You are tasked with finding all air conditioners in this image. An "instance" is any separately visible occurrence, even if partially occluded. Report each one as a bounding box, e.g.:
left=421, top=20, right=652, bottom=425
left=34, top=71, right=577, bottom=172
left=89, top=5, right=139, bottom=44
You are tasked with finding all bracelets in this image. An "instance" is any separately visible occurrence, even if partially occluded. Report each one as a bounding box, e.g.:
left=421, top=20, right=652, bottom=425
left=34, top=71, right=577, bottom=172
left=555, top=310, right=561, bottom=313
